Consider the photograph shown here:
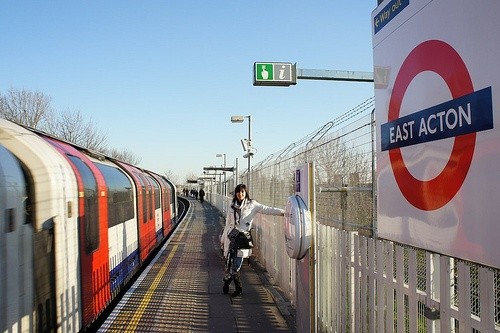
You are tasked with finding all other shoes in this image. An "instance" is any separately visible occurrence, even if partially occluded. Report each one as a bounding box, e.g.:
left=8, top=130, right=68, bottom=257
left=231, top=287, right=243, bottom=297
left=222, top=281, right=230, bottom=294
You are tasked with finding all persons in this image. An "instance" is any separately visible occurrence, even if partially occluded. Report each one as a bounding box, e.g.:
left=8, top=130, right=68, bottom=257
left=219, top=184, right=286, bottom=296
left=183, top=188, right=198, bottom=200
left=199, top=189, right=205, bottom=203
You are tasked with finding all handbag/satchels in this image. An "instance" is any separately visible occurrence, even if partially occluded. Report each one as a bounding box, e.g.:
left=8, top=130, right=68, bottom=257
left=227, top=228, right=246, bottom=245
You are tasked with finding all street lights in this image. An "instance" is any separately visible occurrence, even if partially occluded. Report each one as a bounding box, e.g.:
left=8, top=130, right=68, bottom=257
left=216, top=153, right=227, bottom=195
left=231, top=115, right=251, bottom=196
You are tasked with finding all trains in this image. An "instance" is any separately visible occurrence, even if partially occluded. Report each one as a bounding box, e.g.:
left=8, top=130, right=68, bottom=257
left=0, top=117, right=179, bottom=333
